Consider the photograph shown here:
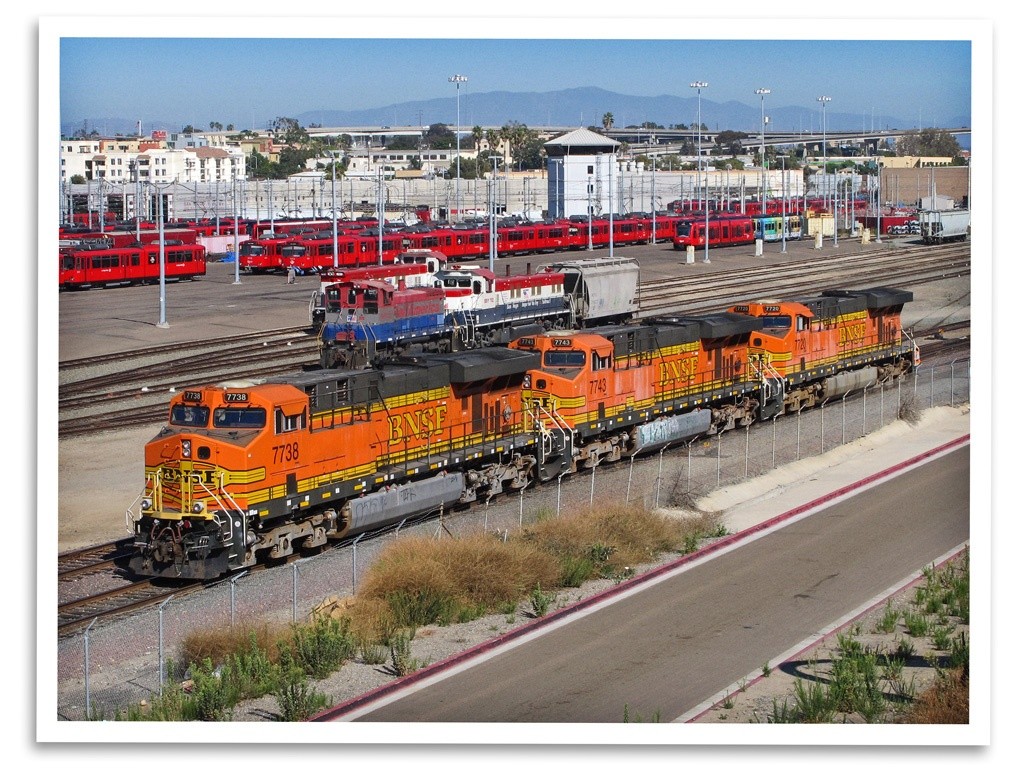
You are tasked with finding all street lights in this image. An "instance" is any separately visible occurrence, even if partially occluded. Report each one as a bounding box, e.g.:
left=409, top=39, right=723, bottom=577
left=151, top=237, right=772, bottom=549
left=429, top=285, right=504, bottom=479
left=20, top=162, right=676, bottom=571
left=690, top=80, right=709, bottom=210
left=755, top=88, right=771, bottom=214
left=447, top=73, right=467, bottom=180
left=817, top=96, right=832, bottom=208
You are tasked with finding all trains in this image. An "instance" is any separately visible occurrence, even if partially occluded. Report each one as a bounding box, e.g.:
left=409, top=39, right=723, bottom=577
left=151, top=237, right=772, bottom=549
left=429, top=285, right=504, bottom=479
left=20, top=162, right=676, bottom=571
left=58, top=201, right=864, bottom=294
left=317, top=257, right=640, bottom=371
left=126, top=284, right=921, bottom=578
